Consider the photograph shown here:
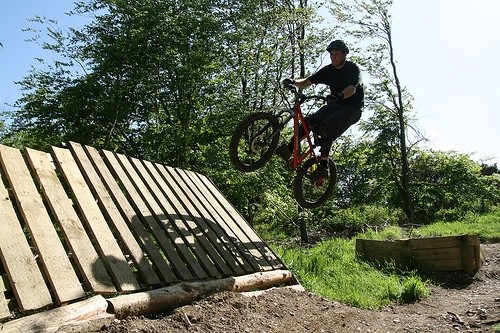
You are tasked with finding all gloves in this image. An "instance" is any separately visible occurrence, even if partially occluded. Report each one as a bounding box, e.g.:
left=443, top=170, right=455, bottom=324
left=283, top=79, right=295, bottom=89
left=323, top=94, right=340, bottom=105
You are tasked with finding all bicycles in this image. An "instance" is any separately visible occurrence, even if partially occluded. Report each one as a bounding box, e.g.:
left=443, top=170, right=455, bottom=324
left=228, top=80, right=338, bottom=210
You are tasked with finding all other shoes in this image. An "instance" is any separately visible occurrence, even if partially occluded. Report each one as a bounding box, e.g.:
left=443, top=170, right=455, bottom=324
left=276, top=143, right=293, bottom=161
left=307, top=164, right=328, bottom=180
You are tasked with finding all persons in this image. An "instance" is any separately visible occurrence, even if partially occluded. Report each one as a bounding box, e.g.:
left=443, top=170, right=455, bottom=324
left=271, top=39, right=365, bottom=180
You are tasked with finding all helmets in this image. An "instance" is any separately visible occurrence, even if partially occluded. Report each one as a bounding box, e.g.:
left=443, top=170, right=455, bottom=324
left=326, top=40, right=349, bottom=54
